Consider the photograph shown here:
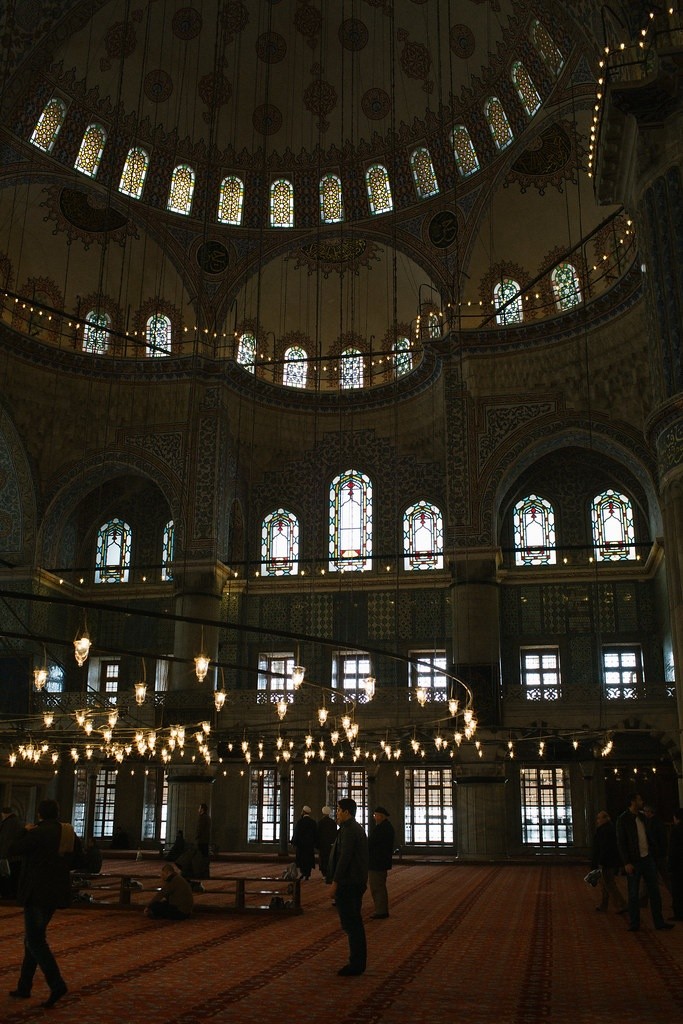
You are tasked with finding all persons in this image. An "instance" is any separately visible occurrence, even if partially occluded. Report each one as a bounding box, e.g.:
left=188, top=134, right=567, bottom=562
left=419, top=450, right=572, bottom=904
left=368, top=808, right=395, bottom=919
left=664, top=808, right=683, bottom=921
left=0, top=806, right=104, bottom=900
left=326, top=797, right=371, bottom=977
left=168, top=804, right=213, bottom=878
left=590, top=810, right=631, bottom=914
left=110, top=827, right=130, bottom=850
left=144, top=864, right=194, bottom=920
left=4, top=798, right=88, bottom=1009
left=292, top=805, right=318, bottom=880
left=316, top=807, right=339, bottom=885
left=616, top=791, right=676, bottom=932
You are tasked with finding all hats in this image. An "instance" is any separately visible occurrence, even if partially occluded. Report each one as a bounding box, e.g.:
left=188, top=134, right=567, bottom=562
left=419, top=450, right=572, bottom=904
left=322, top=807, right=331, bottom=814
left=375, top=807, right=390, bottom=817
left=303, top=806, right=311, bottom=814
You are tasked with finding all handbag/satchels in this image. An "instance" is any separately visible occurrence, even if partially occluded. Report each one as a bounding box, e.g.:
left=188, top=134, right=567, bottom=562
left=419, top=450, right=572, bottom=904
left=584, top=869, right=602, bottom=888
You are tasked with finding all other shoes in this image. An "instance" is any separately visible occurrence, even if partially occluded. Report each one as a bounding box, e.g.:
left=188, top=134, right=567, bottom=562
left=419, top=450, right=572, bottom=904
left=655, top=922, right=675, bottom=930
left=372, top=913, right=389, bottom=917
left=338, top=964, right=361, bottom=976
left=627, top=927, right=639, bottom=932
left=616, top=909, right=628, bottom=915
left=42, top=987, right=68, bottom=1007
left=9, top=990, right=31, bottom=999
left=369, top=914, right=386, bottom=919
left=595, top=907, right=607, bottom=912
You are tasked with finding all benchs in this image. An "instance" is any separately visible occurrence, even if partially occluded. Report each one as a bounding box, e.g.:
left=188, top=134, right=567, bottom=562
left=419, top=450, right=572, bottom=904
left=76, top=872, right=302, bottom=914
